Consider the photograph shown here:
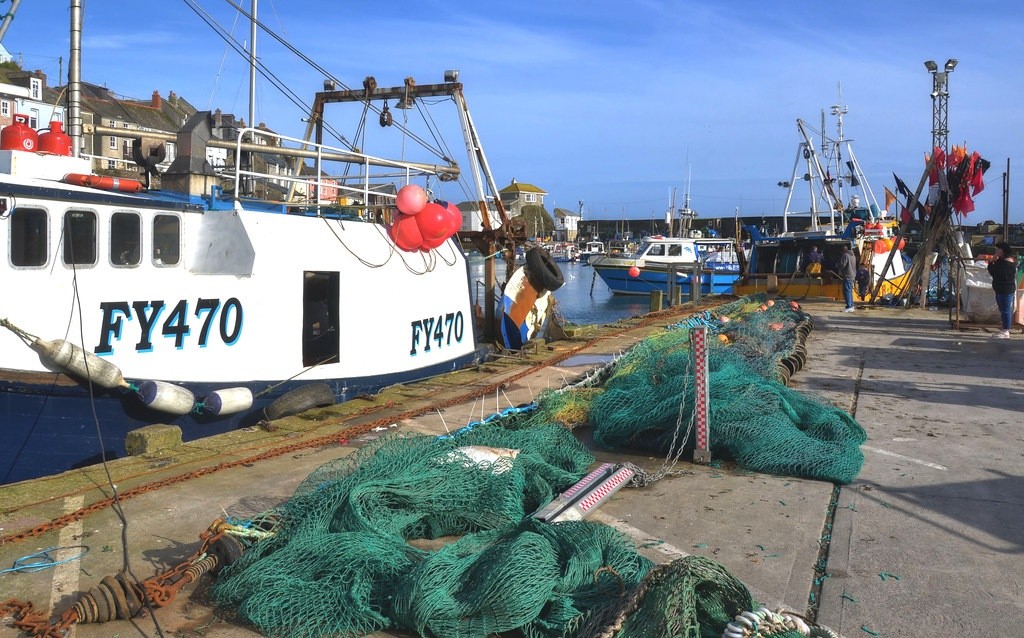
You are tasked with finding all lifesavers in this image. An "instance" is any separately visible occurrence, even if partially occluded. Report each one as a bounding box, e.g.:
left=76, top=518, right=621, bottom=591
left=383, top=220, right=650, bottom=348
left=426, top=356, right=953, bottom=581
left=652, top=236, right=667, bottom=240
left=525, top=246, right=565, bottom=291
left=258, top=381, right=337, bottom=422
left=63, top=172, right=143, bottom=193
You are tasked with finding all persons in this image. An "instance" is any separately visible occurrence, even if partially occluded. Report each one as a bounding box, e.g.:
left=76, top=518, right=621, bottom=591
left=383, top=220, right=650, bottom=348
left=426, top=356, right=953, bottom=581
left=855, top=263, right=870, bottom=301
left=803, top=245, right=824, bottom=279
left=987, top=241, right=1017, bottom=339
left=835, top=242, right=857, bottom=313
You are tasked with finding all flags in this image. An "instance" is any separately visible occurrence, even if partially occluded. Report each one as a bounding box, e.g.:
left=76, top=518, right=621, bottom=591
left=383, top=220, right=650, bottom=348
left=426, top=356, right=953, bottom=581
left=885, top=142, right=991, bottom=224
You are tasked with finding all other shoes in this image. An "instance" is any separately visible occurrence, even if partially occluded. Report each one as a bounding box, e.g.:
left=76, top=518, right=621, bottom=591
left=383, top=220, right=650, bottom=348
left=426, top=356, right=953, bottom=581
left=992, top=329, right=1010, bottom=339
left=840, top=306, right=854, bottom=312
